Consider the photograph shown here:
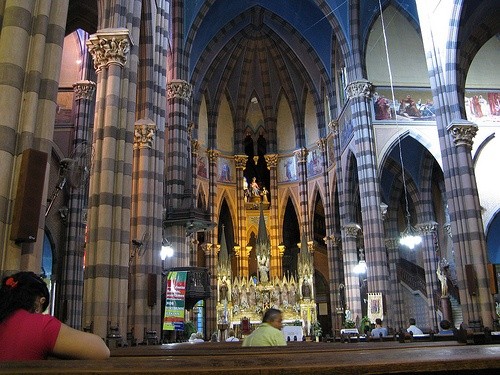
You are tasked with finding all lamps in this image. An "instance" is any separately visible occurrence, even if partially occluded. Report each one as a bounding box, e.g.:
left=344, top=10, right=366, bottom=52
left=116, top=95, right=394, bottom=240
left=379, top=0, right=423, bottom=248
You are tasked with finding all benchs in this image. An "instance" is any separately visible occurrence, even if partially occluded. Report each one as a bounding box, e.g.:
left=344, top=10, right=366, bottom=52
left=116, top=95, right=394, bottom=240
left=0, top=327, right=499, bottom=374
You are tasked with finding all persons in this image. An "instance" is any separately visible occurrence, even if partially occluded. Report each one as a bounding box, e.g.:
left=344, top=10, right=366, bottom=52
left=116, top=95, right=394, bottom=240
left=438, top=271, right=448, bottom=297
left=225, top=331, right=239, bottom=341
left=250, top=177, right=260, bottom=197
left=202, top=327, right=213, bottom=341
left=0, top=271, right=111, bottom=361
left=242, top=177, right=249, bottom=202
left=437, top=320, right=454, bottom=334
left=371, top=318, right=388, bottom=336
left=261, top=187, right=268, bottom=202
left=406, top=318, right=423, bottom=334
left=241, top=308, right=288, bottom=346
